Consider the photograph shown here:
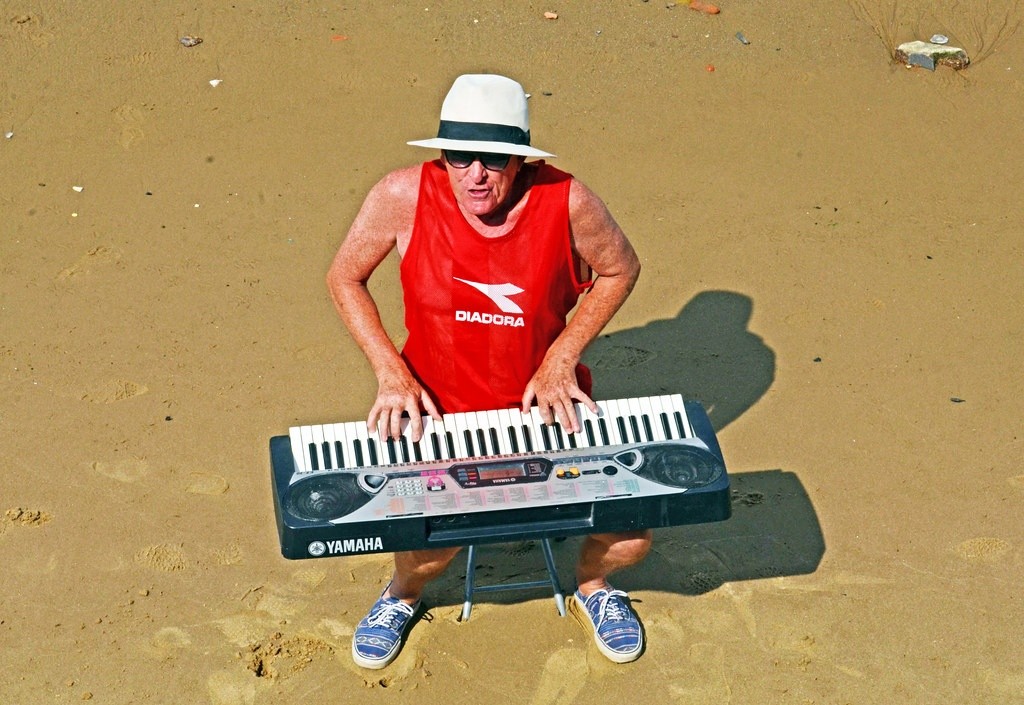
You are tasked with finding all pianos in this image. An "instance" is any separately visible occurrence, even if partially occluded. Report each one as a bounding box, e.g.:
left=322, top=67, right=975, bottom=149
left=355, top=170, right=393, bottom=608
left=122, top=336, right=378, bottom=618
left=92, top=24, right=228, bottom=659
left=267, top=391, right=733, bottom=620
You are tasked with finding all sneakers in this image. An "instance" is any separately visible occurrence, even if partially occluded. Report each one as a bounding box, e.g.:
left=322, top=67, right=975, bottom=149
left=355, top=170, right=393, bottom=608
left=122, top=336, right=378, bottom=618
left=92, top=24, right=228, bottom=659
left=352, top=580, right=421, bottom=669
left=574, top=580, right=644, bottom=663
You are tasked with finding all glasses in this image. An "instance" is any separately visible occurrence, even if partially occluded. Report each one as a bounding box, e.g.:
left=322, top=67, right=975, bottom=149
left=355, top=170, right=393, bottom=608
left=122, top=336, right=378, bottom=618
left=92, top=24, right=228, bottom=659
left=441, top=150, right=512, bottom=171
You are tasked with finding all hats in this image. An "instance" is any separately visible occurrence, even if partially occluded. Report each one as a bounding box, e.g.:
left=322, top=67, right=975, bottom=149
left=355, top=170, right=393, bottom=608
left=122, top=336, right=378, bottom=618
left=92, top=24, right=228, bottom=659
left=407, top=74, right=557, bottom=157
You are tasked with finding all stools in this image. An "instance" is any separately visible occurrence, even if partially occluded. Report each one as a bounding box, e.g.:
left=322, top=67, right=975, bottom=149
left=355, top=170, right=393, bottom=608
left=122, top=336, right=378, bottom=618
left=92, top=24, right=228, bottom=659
left=459, top=364, right=593, bottom=622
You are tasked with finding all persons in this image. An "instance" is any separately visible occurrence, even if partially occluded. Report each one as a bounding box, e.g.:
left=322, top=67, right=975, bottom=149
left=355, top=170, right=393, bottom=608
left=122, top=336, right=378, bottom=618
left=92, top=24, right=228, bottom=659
left=325, top=72, right=653, bottom=670
